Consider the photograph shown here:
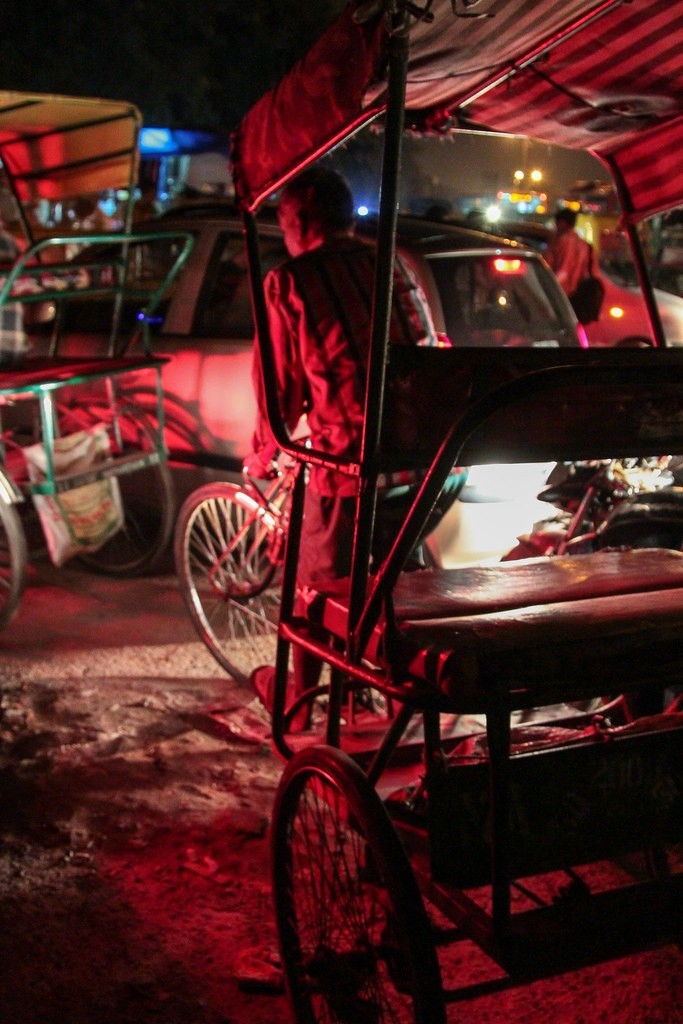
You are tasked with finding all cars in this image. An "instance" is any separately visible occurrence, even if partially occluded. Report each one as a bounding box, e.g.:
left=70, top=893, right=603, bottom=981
left=30, top=201, right=595, bottom=569
left=479, top=215, right=683, bottom=349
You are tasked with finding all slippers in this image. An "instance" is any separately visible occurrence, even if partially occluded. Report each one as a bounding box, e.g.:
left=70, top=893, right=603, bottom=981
left=249, top=666, right=311, bottom=733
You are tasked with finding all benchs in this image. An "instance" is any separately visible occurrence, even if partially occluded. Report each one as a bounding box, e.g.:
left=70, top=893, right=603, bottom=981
left=295, top=345, right=683, bottom=670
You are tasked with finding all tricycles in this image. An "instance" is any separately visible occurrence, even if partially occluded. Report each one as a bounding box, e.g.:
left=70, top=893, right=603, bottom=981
left=0, top=226, right=197, bottom=627
left=171, top=342, right=683, bottom=1024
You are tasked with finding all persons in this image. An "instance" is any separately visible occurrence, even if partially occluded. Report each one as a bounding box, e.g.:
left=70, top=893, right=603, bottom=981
left=240, top=169, right=444, bottom=736
left=540, top=206, right=602, bottom=311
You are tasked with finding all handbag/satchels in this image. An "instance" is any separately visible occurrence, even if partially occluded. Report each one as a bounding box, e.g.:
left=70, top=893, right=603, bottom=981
left=568, top=276, right=605, bottom=324
